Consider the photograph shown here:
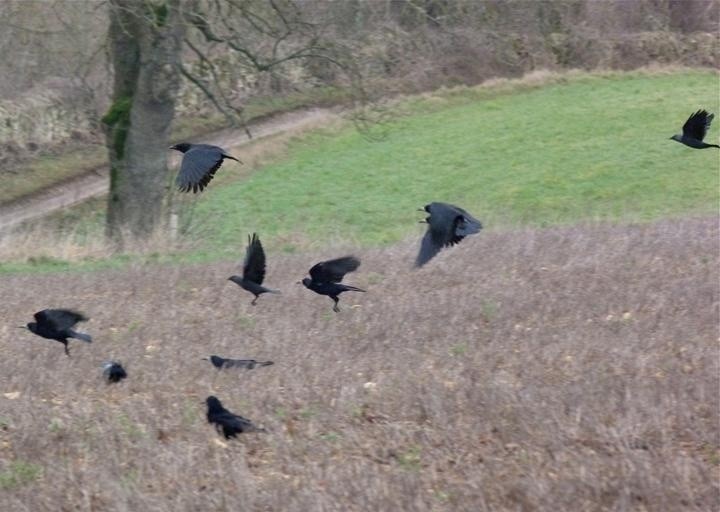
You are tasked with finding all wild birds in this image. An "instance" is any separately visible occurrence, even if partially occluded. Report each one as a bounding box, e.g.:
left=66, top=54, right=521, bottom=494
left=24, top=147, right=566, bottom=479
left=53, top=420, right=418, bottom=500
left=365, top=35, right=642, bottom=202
left=103, top=362, right=127, bottom=384
left=295, top=251, right=369, bottom=314
left=167, top=139, right=244, bottom=195
left=410, top=199, right=484, bottom=272
left=15, top=306, right=96, bottom=361
left=197, top=352, right=280, bottom=373
left=665, top=108, right=720, bottom=151
left=198, top=394, right=267, bottom=443
left=225, top=230, right=282, bottom=309
